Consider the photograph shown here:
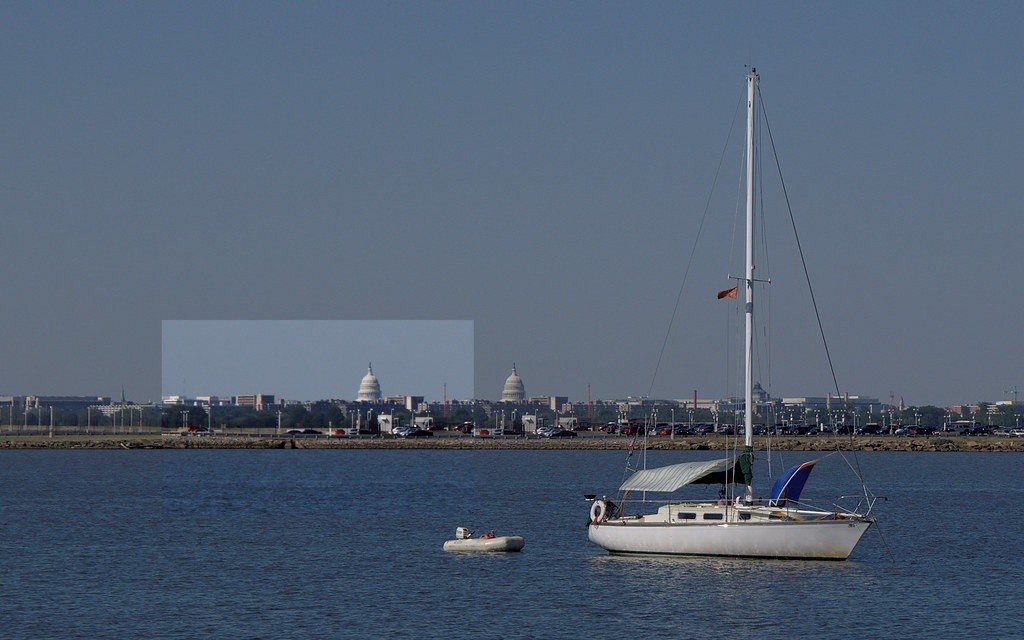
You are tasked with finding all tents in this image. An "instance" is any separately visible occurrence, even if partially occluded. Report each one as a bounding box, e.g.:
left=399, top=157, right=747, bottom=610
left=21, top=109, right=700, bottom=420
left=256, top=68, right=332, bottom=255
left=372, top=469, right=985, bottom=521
left=618, top=457, right=733, bottom=493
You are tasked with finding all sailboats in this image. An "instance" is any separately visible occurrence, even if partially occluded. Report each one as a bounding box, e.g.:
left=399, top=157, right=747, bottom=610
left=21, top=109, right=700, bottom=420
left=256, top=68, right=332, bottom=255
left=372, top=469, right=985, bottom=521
left=583, top=65, right=888, bottom=563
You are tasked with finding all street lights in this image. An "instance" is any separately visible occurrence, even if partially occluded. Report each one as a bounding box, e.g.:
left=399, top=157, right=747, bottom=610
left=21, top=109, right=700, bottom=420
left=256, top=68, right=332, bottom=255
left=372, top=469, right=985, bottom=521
left=494, top=411, right=499, bottom=428
left=535, top=408, right=538, bottom=415
left=179, top=410, right=189, bottom=428
left=553, top=409, right=560, bottom=427
left=570, top=411, right=575, bottom=428
left=49, top=406, right=53, bottom=426
left=391, top=408, right=395, bottom=415
left=276, top=410, right=282, bottom=430
left=425, top=410, right=431, bottom=427
left=349, top=410, right=355, bottom=428
left=409, top=410, right=416, bottom=427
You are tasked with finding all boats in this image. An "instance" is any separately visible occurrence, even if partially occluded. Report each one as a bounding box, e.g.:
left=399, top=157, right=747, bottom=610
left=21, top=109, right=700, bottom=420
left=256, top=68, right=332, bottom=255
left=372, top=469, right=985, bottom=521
left=442, top=527, right=525, bottom=552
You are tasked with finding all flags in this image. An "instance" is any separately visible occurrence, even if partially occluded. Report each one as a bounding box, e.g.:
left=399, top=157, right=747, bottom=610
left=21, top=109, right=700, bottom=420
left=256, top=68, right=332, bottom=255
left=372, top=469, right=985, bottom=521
left=717, top=285, right=738, bottom=300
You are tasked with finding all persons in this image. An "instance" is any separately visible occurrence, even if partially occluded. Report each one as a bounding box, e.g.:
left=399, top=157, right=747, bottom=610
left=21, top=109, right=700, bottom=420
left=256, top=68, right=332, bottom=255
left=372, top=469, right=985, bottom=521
left=717, top=486, right=729, bottom=505
left=479, top=529, right=496, bottom=540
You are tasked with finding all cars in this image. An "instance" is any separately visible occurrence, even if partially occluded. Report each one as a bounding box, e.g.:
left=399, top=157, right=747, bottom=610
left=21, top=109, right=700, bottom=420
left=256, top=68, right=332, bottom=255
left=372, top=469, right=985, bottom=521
left=189, top=425, right=215, bottom=436
left=335, top=428, right=388, bottom=436
left=533, top=426, right=578, bottom=438
left=751, top=405, right=1024, bottom=439
left=282, top=429, right=322, bottom=435
left=598, top=408, right=745, bottom=436
left=388, top=426, right=434, bottom=437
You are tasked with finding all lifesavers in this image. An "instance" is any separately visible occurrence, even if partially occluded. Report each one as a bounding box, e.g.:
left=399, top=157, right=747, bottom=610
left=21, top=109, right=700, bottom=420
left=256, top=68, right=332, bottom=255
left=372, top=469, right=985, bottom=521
left=589, top=499, right=606, bottom=523
